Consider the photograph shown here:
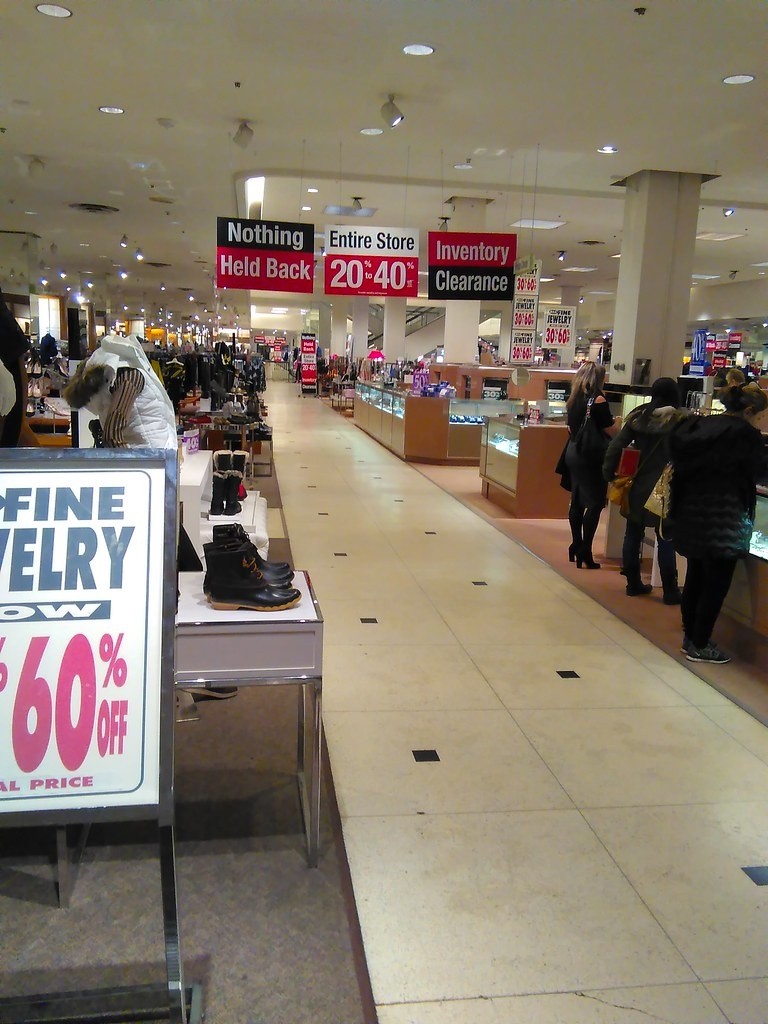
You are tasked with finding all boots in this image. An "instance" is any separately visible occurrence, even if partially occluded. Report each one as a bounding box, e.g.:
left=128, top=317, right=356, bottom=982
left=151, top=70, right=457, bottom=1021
left=214, top=448, right=248, bottom=500
left=202, top=538, right=302, bottom=611
left=88, top=418, right=105, bottom=448
left=210, top=522, right=294, bottom=581
left=210, top=469, right=242, bottom=515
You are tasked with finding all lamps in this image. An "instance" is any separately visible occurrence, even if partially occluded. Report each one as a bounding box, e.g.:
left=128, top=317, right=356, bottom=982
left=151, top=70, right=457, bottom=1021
left=558, top=251, right=565, bottom=261
left=231, top=123, right=256, bottom=146
left=10, top=234, right=166, bottom=309
left=723, top=207, right=734, bottom=217
left=28, top=156, right=45, bottom=181
left=379, top=93, right=406, bottom=130
left=439, top=217, right=449, bottom=232
left=729, top=271, right=737, bottom=280
left=352, top=196, right=363, bottom=213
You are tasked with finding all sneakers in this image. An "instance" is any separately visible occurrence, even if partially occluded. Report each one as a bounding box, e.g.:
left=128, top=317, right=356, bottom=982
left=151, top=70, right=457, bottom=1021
left=686, top=642, right=731, bottom=664
left=680, top=634, right=720, bottom=654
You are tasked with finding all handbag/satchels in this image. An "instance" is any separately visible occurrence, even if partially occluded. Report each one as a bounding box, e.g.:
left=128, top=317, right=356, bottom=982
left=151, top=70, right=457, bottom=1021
left=643, top=461, right=673, bottom=542
left=606, top=472, right=634, bottom=519
left=40, top=333, right=58, bottom=364
left=555, top=434, right=591, bottom=474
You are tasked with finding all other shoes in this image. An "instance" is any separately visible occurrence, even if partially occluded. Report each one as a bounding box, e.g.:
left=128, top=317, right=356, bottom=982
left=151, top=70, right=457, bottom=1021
left=188, top=399, right=273, bottom=441
left=626, top=580, right=652, bottom=596
left=663, top=592, right=684, bottom=605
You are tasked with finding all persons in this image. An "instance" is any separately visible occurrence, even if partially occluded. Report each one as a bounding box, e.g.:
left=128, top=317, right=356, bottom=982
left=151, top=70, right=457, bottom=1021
left=564, top=360, right=614, bottom=569
left=666, top=385, right=768, bottom=663
left=294, top=351, right=301, bottom=383
left=40, top=333, right=58, bottom=367
left=64, top=332, right=238, bottom=698
left=602, top=377, right=693, bottom=605
left=725, top=368, right=748, bottom=387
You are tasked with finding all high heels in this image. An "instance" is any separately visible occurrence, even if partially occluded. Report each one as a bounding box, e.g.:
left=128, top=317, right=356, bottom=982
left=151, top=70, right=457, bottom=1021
left=26, top=397, right=35, bottom=417
left=54, top=361, right=70, bottom=379
left=576, top=543, right=600, bottom=569
left=569, top=542, right=583, bottom=562
left=26, top=360, right=43, bottom=378
left=28, top=377, right=42, bottom=398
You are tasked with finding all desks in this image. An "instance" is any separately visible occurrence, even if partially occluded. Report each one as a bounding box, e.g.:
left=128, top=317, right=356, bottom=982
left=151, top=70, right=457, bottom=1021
left=177, top=570, right=324, bottom=869
